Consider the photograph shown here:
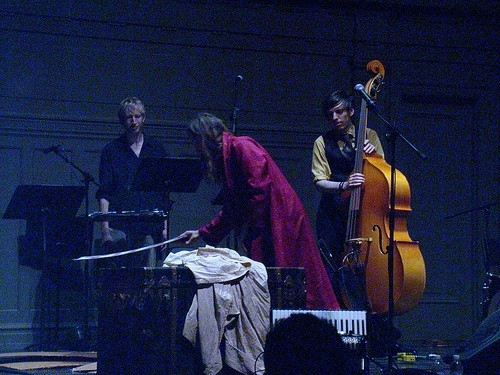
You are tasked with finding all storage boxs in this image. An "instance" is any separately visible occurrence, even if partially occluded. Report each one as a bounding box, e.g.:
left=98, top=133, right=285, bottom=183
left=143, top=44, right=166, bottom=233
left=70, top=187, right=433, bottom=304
left=94, top=266, right=307, bottom=375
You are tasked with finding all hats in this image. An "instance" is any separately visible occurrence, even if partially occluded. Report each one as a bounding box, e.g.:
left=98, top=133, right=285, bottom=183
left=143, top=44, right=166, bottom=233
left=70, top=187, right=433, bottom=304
left=320, top=90, right=349, bottom=112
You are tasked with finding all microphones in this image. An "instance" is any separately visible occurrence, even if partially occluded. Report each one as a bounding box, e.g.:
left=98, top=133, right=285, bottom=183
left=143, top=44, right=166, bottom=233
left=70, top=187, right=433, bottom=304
left=234, top=76, right=243, bottom=90
left=43, top=145, right=61, bottom=154
left=354, top=84, right=381, bottom=114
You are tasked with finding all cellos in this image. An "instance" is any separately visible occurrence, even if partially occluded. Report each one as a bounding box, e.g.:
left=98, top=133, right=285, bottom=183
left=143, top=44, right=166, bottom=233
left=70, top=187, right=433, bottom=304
left=337, top=60, right=426, bottom=318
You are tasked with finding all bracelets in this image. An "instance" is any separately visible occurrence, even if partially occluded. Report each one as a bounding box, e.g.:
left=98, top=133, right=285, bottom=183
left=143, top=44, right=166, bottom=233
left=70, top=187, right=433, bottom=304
left=339, top=181, right=346, bottom=190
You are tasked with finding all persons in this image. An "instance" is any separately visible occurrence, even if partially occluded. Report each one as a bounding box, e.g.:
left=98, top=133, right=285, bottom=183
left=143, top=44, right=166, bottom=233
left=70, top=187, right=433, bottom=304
left=312, top=89, right=386, bottom=264
left=95, top=97, right=170, bottom=260
left=262, top=313, right=362, bottom=375
left=180, top=111, right=340, bottom=310
